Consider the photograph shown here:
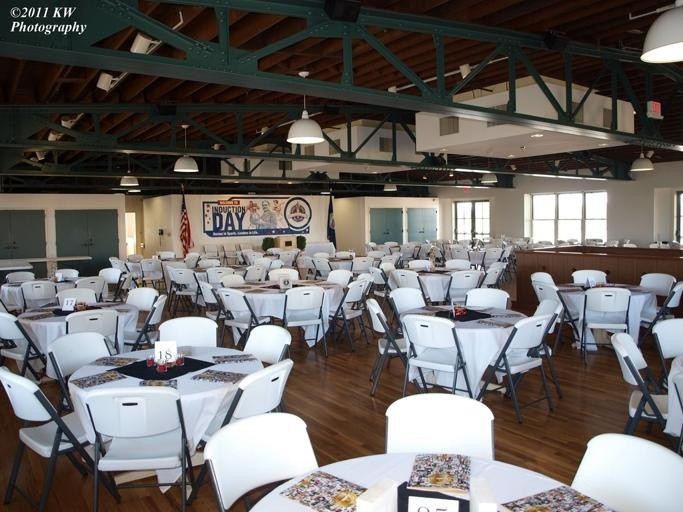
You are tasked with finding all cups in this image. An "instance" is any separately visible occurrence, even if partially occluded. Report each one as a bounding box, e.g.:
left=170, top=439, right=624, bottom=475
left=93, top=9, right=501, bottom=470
left=158, top=360, right=168, bottom=373
left=147, top=354, right=154, bottom=367
left=175, top=355, right=184, bottom=366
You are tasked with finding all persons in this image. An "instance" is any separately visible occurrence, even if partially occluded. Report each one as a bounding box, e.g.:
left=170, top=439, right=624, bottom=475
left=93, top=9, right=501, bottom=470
left=250, top=200, right=278, bottom=230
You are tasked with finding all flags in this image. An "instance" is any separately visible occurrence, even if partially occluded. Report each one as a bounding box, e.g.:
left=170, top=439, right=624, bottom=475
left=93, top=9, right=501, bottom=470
left=326, top=192, right=336, bottom=249
left=180, top=192, right=191, bottom=257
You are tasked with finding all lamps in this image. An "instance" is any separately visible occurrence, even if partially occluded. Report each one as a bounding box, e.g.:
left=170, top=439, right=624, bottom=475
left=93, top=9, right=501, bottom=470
left=640, top=6, right=683, bottom=63
left=286, top=96, right=325, bottom=144
left=630, top=147, right=655, bottom=172
left=481, top=174, right=499, bottom=184
left=173, top=129, right=199, bottom=174
left=128, top=190, right=142, bottom=193
left=47, top=130, right=60, bottom=144
left=36, top=151, right=45, bottom=161
left=384, top=185, right=398, bottom=192
left=120, top=156, right=140, bottom=187
left=96, top=72, right=113, bottom=92
left=60, top=117, right=75, bottom=128
left=129, top=31, right=158, bottom=54
left=459, top=63, right=471, bottom=79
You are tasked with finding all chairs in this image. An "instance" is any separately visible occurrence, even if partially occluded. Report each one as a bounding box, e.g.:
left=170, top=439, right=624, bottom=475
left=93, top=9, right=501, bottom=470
left=184, top=252, right=200, bottom=267
left=199, top=280, right=230, bottom=323
left=222, top=244, right=254, bottom=266
left=531, top=271, right=557, bottom=287
left=671, top=370, right=683, bottom=455
left=366, top=299, right=422, bottom=395
left=0, top=298, right=11, bottom=314
left=217, top=287, right=272, bottom=347
left=203, top=413, right=320, bottom=512
left=207, top=267, right=234, bottom=284
left=504, top=299, right=564, bottom=400
left=444, top=232, right=519, bottom=266
left=322, top=279, right=372, bottom=352
left=109, top=255, right=138, bottom=287
left=0, top=366, right=122, bottom=512
left=385, top=392, right=496, bottom=460
left=581, top=287, right=631, bottom=365
left=243, top=325, right=292, bottom=364
left=570, top=433, right=683, bottom=512
left=254, top=242, right=302, bottom=267
left=475, top=312, right=558, bottom=423
left=56, top=288, right=97, bottom=305
left=124, top=295, right=168, bottom=351
left=6, top=271, right=35, bottom=285
left=244, top=266, right=267, bottom=282
left=326, top=270, right=354, bottom=284
left=159, top=316, right=219, bottom=348
left=652, top=318, right=683, bottom=394
left=311, top=257, right=333, bottom=280
left=66, top=310, right=123, bottom=355
left=521, top=238, right=682, bottom=249
left=351, top=255, right=373, bottom=271
left=278, top=286, right=329, bottom=358
left=482, top=267, right=504, bottom=288
left=140, top=259, right=166, bottom=290
left=611, top=332, right=677, bottom=449
left=171, top=268, right=207, bottom=319
left=202, top=245, right=221, bottom=258
left=357, top=273, right=374, bottom=296
left=391, top=270, right=432, bottom=305
left=269, top=268, right=300, bottom=281
left=75, top=276, right=106, bottom=303
left=186, top=359, right=293, bottom=506
left=20, top=281, right=56, bottom=309
left=0, top=312, right=46, bottom=377
left=369, top=266, right=391, bottom=309
left=465, top=288, right=510, bottom=310
left=198, top=259, right=220, bottom=268
left=402, top=314, right=474, bottom=398
left=220, top=274, right=245, bottom=286
left=409, top=260, right=432, bottom=269
left=54, top=269, right=79, bottom=282
left=532, top=281, right=580, bottom=355
left=389, top=287, right=425, bottom=314
left=368, top=240, right=406, bottom=266
left=639, top=272, right=676, bottom=296
left=126, top=287, right=159, bottom=331
left=156, top=250, right=176, bottom=261
left=80, top=386, right=196, bottom=512
left=406, top=240, right=444, bottom=260
left=638, top=281, right=683, bottom=348
left=46, top=332, right=112, bottom=410
left=303, top=257, right=322, bottom=281
left=106, top=271, right=133, bottom=302
left=571, top=269, right=607, bottom=286
left=302, top=240, right=355, bottom=256
left=98, top=268, right=122, bottom=283
left=444, top=272, right=489, bottom=305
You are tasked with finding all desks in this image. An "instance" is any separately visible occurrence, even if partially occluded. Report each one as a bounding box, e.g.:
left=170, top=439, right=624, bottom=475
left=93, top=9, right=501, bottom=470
left=1, top=258, right=33, bottom=270
left=28, top=255, right=93, bottom=262
